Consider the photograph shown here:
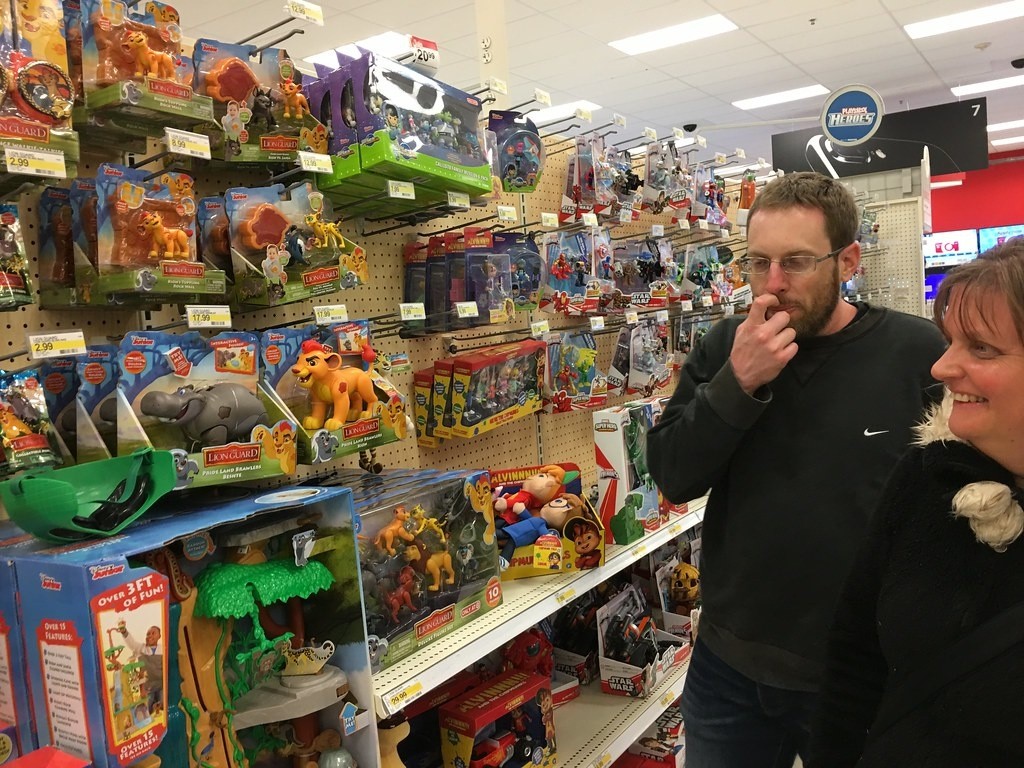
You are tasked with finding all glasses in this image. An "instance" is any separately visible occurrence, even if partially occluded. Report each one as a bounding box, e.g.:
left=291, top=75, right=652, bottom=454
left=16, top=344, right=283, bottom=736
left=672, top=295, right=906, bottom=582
left=735, top=248, right=847, bottom=274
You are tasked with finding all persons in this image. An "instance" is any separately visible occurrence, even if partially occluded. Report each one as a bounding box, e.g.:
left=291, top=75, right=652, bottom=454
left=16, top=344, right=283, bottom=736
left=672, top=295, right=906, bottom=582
left=510, top=707, right=533, bottom=742
left=222, top=100, right=245, bottom=141
left=117, top=620, right=164, bottom=715
left=800, top=236, right=1023, bottom=767
left=491, top=464, right=593, bottom=573
left=645, top=172, right=950, bottom=768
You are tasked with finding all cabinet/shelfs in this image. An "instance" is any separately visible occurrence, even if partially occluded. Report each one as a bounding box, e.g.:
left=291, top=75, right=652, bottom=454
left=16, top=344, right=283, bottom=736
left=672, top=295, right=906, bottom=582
left=370, top=497, right=709, bottom=768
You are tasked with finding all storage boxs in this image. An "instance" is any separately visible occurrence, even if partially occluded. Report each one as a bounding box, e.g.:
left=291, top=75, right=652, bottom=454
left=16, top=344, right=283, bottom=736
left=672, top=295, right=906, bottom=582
left=438, top=669, right=558, bottom=768
left=592, top=395, right=689, bottom=546
left=609, top=693, right=686, bottom=768
left=413, top=339, right=546, bottom=450
left=277, top=467, right=503, bottom=677
left=300, top=42, right=493, bottom=220
left=0, top=487, right=381, bottom=768
